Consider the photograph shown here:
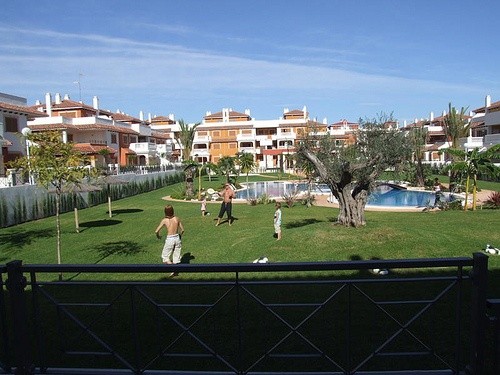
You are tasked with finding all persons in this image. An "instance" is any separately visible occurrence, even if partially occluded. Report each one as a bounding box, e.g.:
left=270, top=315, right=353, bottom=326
left=273, top=202, right=282, bottom=241
left=432, top=178, right=442, bottom=192
left=214, top=183, right=236, bottom=227
left=200, top=197, right=206, bottom=216
left=155, top=205, right=184, bottom=278
